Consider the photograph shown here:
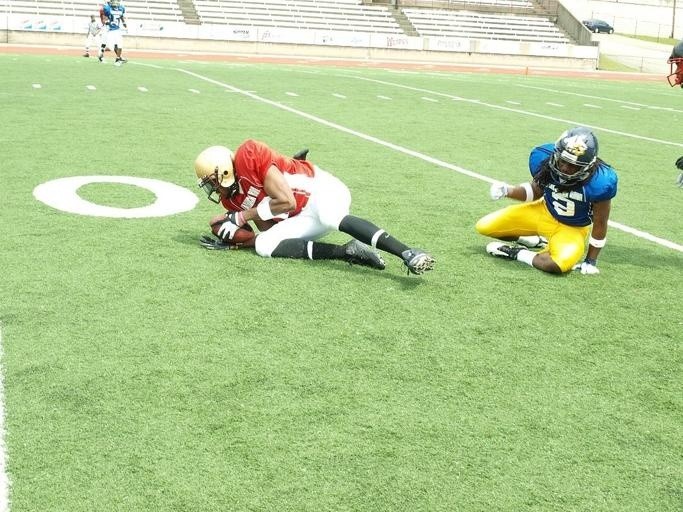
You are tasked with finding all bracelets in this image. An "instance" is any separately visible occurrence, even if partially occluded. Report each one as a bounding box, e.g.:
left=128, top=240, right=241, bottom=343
left=584, top=257, right=596, bottom=266
left=239, top=211, right=247, bottom=224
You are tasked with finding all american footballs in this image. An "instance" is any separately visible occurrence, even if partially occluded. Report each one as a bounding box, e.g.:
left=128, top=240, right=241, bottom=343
left=210, top=213, right=255, bottom=243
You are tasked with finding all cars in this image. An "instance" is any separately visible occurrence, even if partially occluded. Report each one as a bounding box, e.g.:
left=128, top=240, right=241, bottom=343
left=583, top=19, right=614, bottom=34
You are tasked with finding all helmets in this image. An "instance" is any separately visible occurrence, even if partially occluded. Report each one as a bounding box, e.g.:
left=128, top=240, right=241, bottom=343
left=194, top=145, right=239, bottom=204
left=105, top=0, right=121, bottom=7
left=545, top=127, right=599, bottom=189
left=666, top=41, right=683, bottom=88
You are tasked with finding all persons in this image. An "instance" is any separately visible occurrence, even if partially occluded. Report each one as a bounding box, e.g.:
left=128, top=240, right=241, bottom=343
left=196, top=139, right=436, bottom=276
left=83, top=0, right=128, bottom=64
left=475, top=127, right=618, bottom=276
left=669, top=40, right=683, bottom=188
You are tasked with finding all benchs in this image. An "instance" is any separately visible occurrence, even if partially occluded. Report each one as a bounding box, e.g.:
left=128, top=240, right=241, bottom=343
left=0, top=0, right=571, bottom=45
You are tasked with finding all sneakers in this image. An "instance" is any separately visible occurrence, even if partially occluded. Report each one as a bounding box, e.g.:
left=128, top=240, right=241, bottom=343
left=486, top=240, right=528, bottom=261
left=532, top=236, right=549, bottom=249
left=343, top=237, right=386, bottom=270
left=82, top=52, right=128, bottom=65
left=399, top=248, right=436, bottom=276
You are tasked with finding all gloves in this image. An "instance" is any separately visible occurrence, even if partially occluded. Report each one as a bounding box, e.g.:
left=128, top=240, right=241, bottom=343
left=674, top=157, right=682, bottom=189
left=210, top=210, right=242, bottom=243
left=490, top=182, right=508, bottom=201
left=572, top=258, right=600, bottom=275
left=198, top=231, right=239, bottom=251
